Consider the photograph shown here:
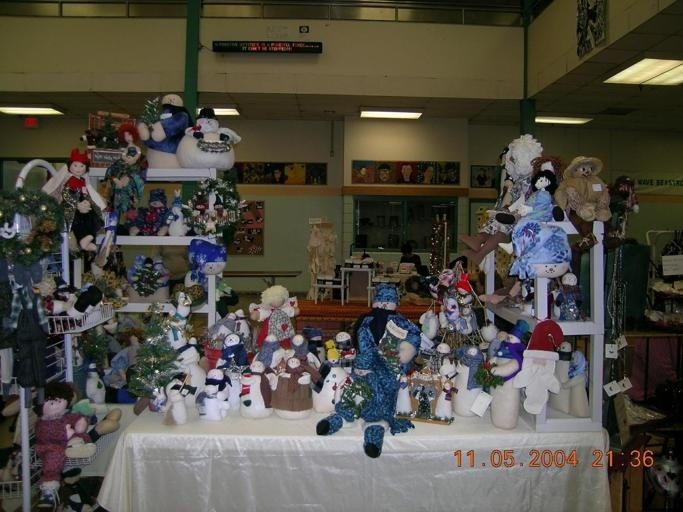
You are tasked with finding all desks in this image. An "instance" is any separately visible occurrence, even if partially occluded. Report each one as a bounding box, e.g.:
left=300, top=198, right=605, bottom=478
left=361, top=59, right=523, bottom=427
left=95, top=397, right=614, bottom=512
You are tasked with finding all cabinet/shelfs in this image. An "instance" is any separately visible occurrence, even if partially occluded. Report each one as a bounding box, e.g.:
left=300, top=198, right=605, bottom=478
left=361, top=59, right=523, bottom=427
left=73, top=166, right=223, bottom=367
left=307, top=266, right=402, bottom=309
left=623, top=330, right=683, bottom=403
left=484, top=220, right=605, bottom=432
left=0, top=215, right=73, bottom=512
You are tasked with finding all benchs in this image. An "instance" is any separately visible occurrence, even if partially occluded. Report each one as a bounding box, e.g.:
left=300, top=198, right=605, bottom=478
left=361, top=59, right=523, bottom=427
left=220, top=270, right=300, bottom=286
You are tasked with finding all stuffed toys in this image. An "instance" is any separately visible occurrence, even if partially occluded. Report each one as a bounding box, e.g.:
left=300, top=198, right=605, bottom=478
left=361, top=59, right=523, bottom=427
left=0, top=91, right=639, bottom=496
left=0, top=91, right=640, bottom=495
